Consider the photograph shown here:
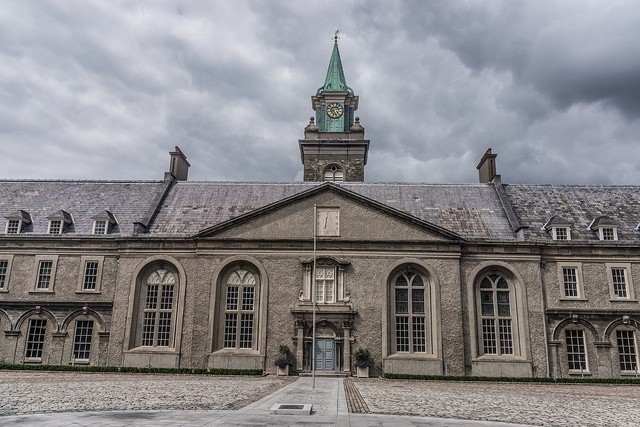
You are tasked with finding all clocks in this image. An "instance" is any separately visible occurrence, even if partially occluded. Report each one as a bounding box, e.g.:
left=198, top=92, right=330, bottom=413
left=326, top=102, right=343, bottom=118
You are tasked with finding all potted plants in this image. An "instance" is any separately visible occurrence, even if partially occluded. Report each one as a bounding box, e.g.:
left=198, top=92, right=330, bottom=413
left=278, top=345, right=289, bottom=360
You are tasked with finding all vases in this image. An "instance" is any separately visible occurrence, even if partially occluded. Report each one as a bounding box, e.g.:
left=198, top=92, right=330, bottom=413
left=277, top=364, right=289, bottom=376
left=357, top=366, right=369, bottom=378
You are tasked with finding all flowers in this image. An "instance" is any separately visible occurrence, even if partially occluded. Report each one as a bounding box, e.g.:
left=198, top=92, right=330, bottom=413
left=273, top=358, right=288, bottom=369
left=354, top=360, right=371, bottom=367
